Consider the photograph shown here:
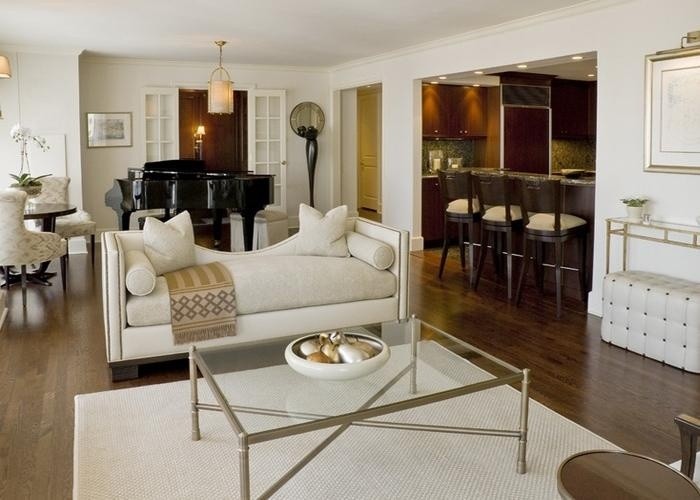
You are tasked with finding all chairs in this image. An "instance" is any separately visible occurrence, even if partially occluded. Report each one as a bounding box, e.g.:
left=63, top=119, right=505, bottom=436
left=33, top=177, right=97, bottom=295
left=439, top=171, right=589, bottom=322
left=0, top=190, right=70, bottom=309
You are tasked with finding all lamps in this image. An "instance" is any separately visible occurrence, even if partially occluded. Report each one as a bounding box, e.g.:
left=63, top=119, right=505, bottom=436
left=196, top=125, right=206, bottom=140
left=208, top=40, right=234, bottom=115
left=0, top=56, right=12, bottom=120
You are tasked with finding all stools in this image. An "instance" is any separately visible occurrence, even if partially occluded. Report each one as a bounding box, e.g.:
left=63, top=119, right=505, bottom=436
left=230, top=212, right=288, bottom=252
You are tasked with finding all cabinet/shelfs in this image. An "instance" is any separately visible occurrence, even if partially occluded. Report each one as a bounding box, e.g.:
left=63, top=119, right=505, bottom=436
left=422, top=178, right=457, bottom=240
left=422, top=82, right=488, bottom=139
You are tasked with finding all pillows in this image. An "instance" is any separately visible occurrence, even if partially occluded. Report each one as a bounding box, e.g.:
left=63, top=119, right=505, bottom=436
left=143, top=211, right=197, bottom=276
left=345, top=231, right=396, bottom=271
left=297, top=202, right=350, bottom=258
left=124, top=249, right=156, bottom=298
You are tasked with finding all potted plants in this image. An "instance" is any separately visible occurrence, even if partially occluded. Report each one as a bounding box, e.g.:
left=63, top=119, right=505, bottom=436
left=8, top=174, right=52, bottom=196
left=620, top=197, right=648, bottom=221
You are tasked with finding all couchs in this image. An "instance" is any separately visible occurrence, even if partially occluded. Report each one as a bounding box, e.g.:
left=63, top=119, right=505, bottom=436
left=100, top=205, right=412, bottom=381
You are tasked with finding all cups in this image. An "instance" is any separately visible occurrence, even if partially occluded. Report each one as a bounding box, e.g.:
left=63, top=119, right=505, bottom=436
left=434, top=159, right=441, bottom=170
left=452, top=164, right=458, bottom=169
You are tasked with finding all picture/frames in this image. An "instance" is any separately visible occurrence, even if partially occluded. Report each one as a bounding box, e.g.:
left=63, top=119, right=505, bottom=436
left=87, top=111, right=132, bottom=148
left=644, top=49, right=700, bottom=174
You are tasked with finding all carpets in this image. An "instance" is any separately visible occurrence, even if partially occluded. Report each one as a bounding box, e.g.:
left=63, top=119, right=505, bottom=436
left=71, top=337, right=628, bottom=500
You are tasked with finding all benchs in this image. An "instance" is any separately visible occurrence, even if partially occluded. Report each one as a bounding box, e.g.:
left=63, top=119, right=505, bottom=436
left=601, top=270, right=700, bottom=374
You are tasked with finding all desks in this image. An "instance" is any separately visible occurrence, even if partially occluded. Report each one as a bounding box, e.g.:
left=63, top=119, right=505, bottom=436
left=0, top=202, right=76, bottom=289
left=557, top=450, right=700, bottom=500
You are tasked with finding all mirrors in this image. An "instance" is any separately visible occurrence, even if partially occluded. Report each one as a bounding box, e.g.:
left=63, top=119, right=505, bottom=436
left=290, top=101, right=325, bottom=139
